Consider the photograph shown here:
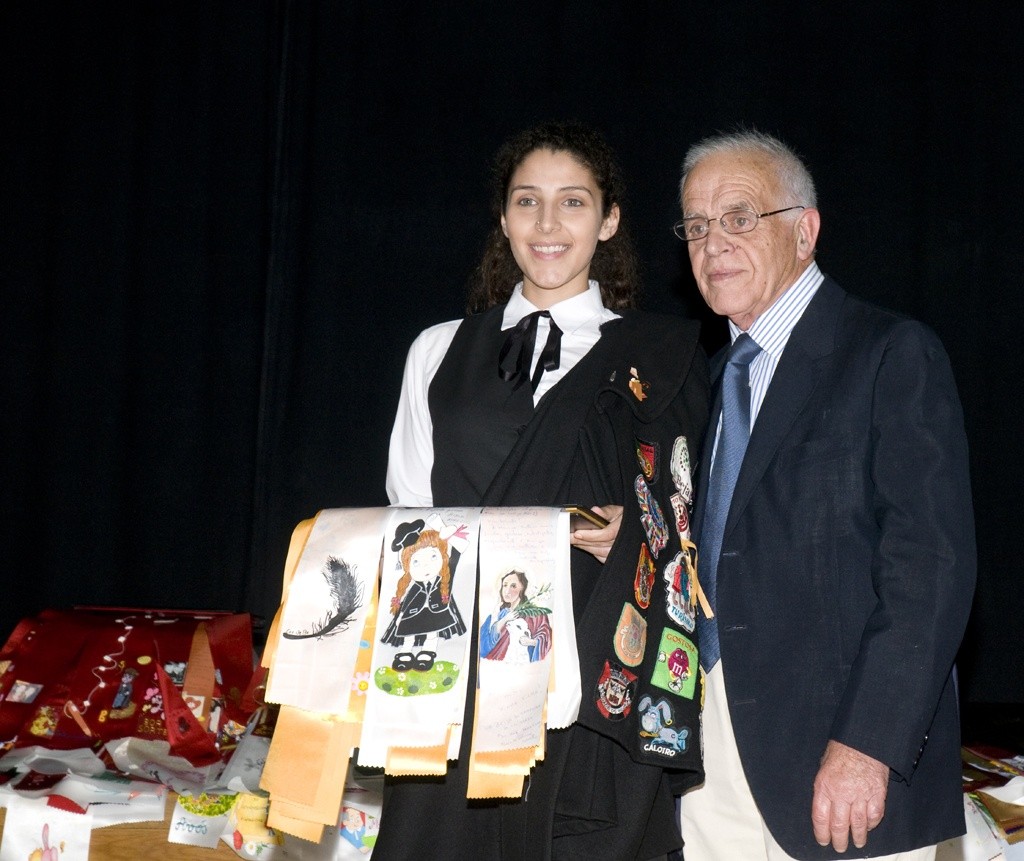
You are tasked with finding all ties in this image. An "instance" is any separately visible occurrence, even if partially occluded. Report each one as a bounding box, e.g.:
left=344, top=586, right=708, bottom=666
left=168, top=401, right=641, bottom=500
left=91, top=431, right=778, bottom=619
left=695, top=333, right=763, bottom=674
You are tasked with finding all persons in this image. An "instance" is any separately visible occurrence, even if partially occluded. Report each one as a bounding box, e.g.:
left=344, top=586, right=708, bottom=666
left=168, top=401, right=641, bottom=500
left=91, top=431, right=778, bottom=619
left=670, top=137, right=980, bottom=861
left=369, top=127, right=701, bottom=861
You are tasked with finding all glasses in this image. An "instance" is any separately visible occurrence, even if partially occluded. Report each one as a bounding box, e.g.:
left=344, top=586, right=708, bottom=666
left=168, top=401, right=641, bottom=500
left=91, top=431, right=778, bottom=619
left=672, top=206, right=806, bottom=242
